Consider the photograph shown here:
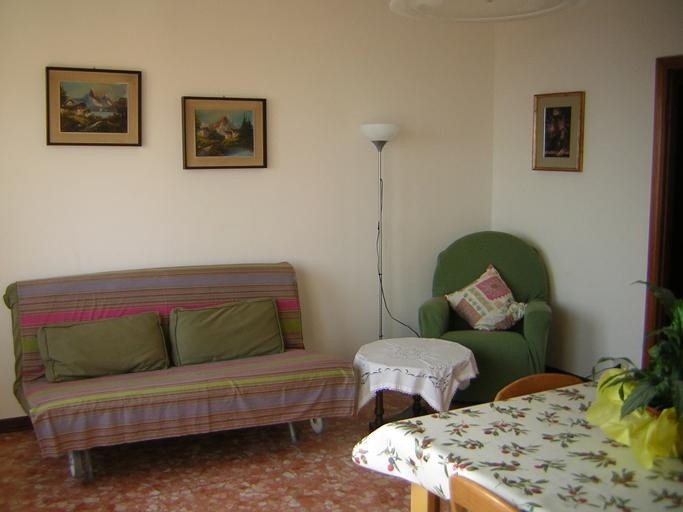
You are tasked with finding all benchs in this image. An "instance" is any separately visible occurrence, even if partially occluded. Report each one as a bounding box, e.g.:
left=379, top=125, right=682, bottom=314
left=3, top=262, right=359, bottom=479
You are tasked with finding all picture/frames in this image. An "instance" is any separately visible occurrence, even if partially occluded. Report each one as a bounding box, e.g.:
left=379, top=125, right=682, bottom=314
left=45, top=65, right=143, bottom=146
left=532, top=90, right=587, bottom=173
left=181, top=96, right=268, bottom=169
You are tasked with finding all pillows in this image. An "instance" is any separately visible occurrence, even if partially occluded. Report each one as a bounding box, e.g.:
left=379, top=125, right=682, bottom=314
left=36, top=310, right=171, bottom=384
left=165, top=294, right=285, bottom=368
left=442, top=263, right=527, bottom=333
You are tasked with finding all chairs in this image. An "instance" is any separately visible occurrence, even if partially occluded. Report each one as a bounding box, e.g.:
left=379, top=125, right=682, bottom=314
left=492, top=374, right=584, bottom=402
left=443, top=472, right=524, bottom=511
left=417, top=230, right=554, bottom=402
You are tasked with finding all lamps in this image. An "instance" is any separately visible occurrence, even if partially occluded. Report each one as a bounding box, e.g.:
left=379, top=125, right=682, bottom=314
left=361, top=123, right=398, bottom=430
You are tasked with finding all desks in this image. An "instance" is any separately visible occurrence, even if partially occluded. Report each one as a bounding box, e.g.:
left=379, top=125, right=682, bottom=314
left=352, top=375, right=681, bottom=512
left=350, top=335, right=479, bottom=428
